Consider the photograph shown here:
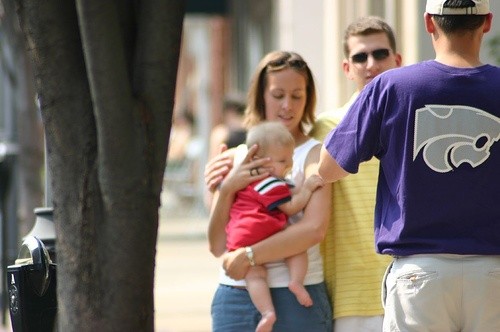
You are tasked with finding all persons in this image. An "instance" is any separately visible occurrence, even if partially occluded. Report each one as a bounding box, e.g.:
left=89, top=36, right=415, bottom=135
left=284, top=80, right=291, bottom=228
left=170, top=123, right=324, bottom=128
left=165, top=100, right=247, bottom=192
left=204, top=15, right=404, bottom=332
left=225, top=122, right=326, bottom=332
left=318, top=0, right=500, bottom=332
left=208, top=50, right=335, bottom=332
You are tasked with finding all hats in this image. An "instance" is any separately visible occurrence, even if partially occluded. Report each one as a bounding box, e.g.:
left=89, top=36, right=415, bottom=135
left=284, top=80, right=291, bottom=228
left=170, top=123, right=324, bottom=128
left=426, top=1, right=489, bottom=15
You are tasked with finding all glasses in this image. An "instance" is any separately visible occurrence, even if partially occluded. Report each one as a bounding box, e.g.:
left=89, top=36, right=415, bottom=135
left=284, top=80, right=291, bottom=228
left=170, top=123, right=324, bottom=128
left=266, top=60, right=306, bottom=72
left=350, top=48, right=390, bottom=64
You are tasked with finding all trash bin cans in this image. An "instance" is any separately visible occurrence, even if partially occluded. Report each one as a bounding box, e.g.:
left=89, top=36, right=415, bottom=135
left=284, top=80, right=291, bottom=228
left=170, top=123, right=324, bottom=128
left=0, top=237, right=59, bottom=330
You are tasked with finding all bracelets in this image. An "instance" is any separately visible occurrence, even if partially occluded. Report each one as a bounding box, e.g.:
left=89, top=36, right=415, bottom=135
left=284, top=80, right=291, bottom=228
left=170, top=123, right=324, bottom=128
left=243, top=246, right=258, bottom=270
